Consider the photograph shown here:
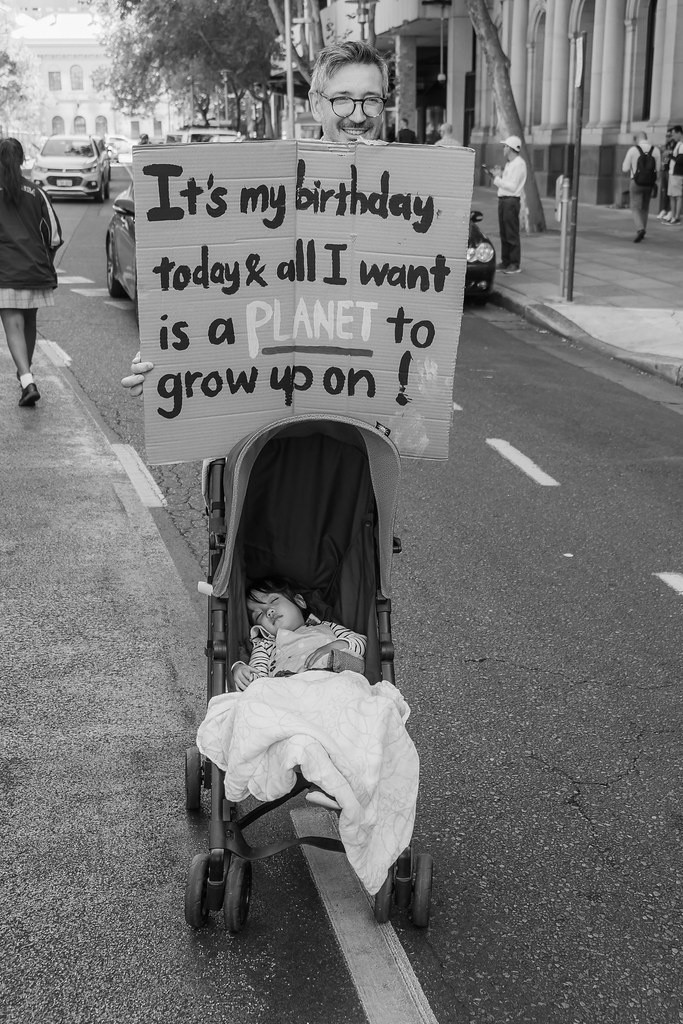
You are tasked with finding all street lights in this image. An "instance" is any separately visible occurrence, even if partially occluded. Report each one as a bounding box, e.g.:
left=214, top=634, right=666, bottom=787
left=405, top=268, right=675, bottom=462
left=187, top=75, right=196, bottom=127
left=219, top=69, right=232, bottom=121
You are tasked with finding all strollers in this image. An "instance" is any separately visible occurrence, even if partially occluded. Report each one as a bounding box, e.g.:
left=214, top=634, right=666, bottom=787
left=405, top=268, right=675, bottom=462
left=183, top=414, right=434, bottom=931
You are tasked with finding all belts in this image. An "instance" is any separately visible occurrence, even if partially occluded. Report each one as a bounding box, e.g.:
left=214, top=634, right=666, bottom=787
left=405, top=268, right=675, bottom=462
left=498, top=196, right=520, bottom=199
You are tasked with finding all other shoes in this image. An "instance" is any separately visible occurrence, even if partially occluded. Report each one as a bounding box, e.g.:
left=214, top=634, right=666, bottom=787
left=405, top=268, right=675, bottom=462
left=656, top=209, right=667, bottom=219
left=16, top=370, right=21, bottom=381
left=669, top=217, right=680, bottom=223
left=503, top=263, right=521, bottom=274
left=495, top=263, right=506, bottom=272
left=633, top=230, right=646, bottom=243
left=19, top=383, right=41, bottom=406
left=663, top=211, right=674, bottom=221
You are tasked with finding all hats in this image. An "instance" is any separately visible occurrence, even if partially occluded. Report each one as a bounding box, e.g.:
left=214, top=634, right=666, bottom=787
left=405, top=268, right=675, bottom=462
left=500, top=135, right=521, bottom=152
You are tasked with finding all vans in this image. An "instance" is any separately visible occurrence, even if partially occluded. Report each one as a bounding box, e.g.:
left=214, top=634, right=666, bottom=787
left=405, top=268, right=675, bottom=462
left=164, top=130, right=244, bottom=145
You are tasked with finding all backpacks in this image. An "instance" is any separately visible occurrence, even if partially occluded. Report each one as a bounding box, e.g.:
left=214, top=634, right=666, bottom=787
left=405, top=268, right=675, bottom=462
left=630, top=145, right=657, bottom=187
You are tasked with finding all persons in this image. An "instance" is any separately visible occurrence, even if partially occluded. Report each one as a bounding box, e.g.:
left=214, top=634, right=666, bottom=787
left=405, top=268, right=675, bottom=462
left=138, top=134, right=151, bottom=145
left=657, top=125, right=683, bottom=223
left=120, top=41, right=389, bottom=401
left=397, top=118, right=416, bottom=144
left=486, top=136, right=527, bottom=273
left=426, top=121, right=461, bottom=147
left=0, top=138, right=64, bottom=404
left=232, top=580, right=367, bottom=810
left=622, top=131, right=661, bottom=243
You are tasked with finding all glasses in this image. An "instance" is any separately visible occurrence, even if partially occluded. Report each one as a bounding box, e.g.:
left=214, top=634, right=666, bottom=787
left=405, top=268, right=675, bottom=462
left=503, top=145, right=510, bottom=148
left=665, top=134, right=672, bottom=138
left=315, top=89, right=388, bottom=118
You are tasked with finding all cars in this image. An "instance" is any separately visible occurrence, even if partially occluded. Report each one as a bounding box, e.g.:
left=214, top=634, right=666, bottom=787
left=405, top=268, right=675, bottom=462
left=465, top=210, right=496, bottom=310
left=22, top=133, right=133, bottom=202
left=106, top=180, right=139, bottom=327
